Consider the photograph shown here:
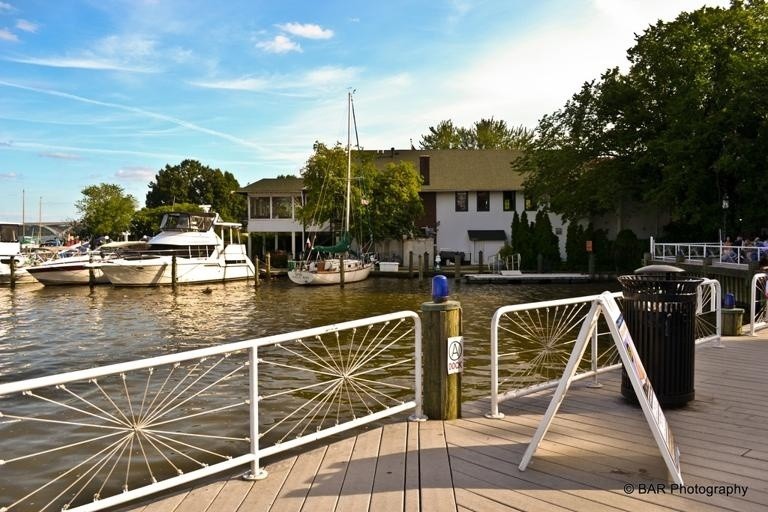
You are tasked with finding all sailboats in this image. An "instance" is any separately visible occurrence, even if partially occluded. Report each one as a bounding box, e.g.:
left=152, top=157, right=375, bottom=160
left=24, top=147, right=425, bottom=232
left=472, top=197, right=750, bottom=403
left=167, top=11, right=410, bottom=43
left=288, top=88, right=378, bottom=284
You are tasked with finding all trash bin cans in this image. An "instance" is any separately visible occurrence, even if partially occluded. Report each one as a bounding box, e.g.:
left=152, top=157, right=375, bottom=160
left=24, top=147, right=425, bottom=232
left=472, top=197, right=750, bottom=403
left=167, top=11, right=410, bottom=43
left=21, top=236, right=31, bottom=252
left=619, top=276, right=698, bottom=409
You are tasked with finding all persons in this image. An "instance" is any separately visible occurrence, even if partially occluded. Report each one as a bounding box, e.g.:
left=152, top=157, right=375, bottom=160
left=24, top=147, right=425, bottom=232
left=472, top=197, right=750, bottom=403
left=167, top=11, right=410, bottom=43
left=722, top=235, right=768, bottom=264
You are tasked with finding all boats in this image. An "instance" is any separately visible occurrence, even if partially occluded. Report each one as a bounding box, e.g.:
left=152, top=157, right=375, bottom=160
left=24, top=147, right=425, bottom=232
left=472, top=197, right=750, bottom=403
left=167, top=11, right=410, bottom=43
left=0, top=190, right=256, bottom=285
